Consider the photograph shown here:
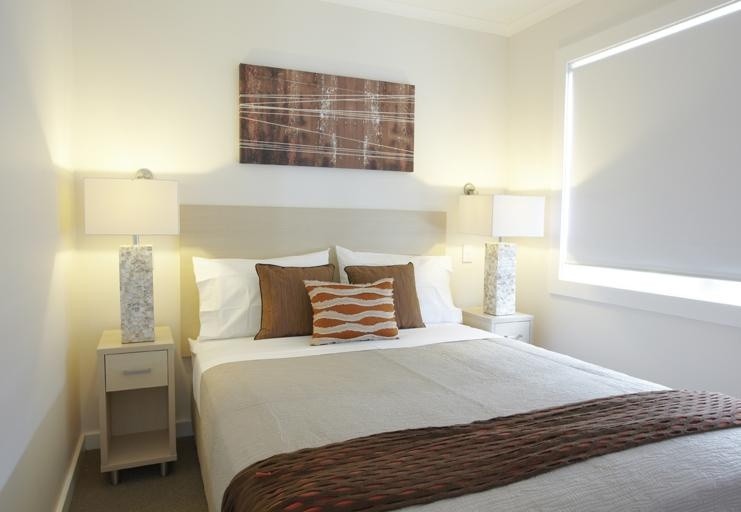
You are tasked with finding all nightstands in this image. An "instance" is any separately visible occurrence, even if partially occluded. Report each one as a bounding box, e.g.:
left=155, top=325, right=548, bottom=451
left=461, top=308, right=534, bottom=345
left=96, top=327, right=179, bottom=486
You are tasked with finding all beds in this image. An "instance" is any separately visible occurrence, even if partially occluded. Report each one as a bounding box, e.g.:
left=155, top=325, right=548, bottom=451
left=180, top=205, right=741, bottom=512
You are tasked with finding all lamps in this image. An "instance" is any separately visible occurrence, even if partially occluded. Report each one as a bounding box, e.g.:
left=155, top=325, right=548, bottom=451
left=459, top=194, right=546, bottom=316
left=82, top=178, right=181, bottom=344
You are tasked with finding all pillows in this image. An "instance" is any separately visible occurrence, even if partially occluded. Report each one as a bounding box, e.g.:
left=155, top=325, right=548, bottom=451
left=191, top=245, right=464, bottom=346
